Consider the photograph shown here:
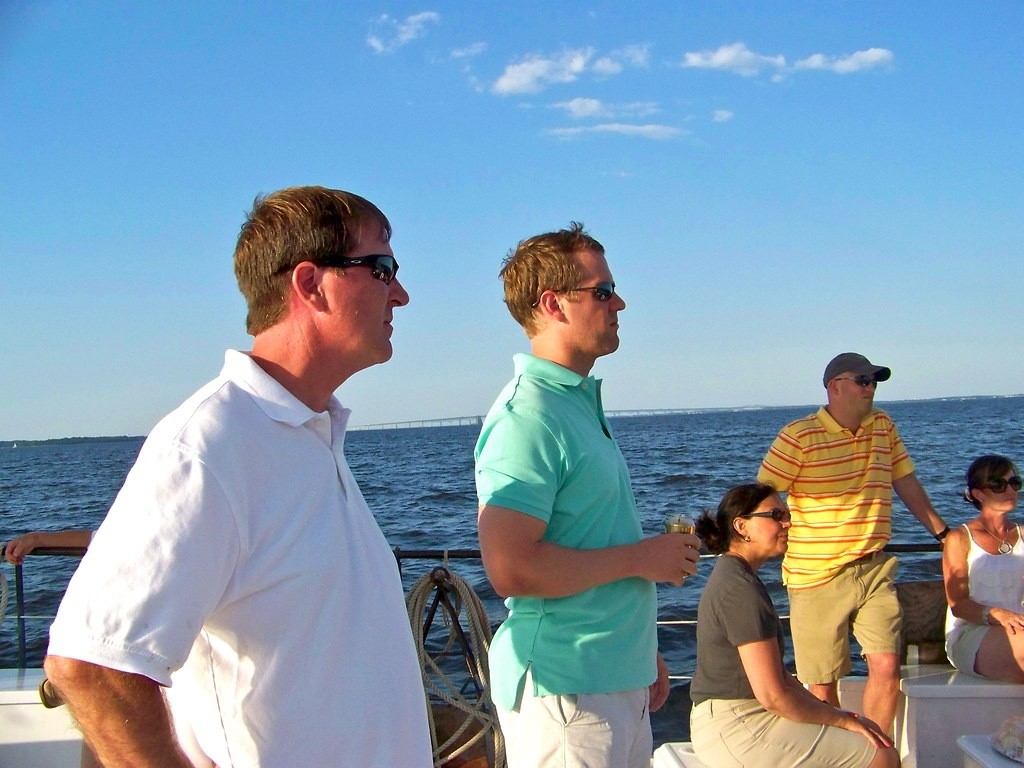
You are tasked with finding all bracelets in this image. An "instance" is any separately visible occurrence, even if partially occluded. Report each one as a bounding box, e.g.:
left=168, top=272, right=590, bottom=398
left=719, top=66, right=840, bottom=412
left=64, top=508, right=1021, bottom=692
left=935, top=525, right=951, bottom=541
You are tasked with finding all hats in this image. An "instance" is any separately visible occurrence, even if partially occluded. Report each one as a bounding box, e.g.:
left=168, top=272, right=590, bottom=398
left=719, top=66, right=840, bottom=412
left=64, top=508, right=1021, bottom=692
left=823, top=352, right=891, bottom=389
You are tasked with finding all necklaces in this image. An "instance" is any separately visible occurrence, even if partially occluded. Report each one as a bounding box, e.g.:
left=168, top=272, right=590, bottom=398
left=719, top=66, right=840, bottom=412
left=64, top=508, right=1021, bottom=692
left=976, top=518, right=1013, bottom=556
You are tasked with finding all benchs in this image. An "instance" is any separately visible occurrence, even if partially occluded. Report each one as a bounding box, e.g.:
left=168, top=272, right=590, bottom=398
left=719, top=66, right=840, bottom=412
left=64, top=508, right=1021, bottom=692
left=893, top=580, right=1024, bottom=768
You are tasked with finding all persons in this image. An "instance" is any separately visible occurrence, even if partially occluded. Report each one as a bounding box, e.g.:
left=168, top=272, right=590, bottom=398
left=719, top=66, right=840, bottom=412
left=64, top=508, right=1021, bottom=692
left=942, top=454, right=1024, bottom=685
left=688, top=481, right=902, bottom=768
left=43, top=185, right=434, bottom=768
left=755, top=353, right=952, bottom=740
left=473, top=222, right=703, bottom=767
left=5, top=530, right=98, bottom=565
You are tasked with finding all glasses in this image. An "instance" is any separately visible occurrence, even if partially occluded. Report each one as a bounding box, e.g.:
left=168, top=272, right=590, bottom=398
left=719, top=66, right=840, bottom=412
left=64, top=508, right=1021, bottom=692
left=835, top=375, right=877, bottom=389
left=741, top=509, right=791, bottom=522
left=981, top=475, right=1022, bottom=494
left=275, top=254, right=399, bottom=286
left=532, top=281, right=616, bottom=307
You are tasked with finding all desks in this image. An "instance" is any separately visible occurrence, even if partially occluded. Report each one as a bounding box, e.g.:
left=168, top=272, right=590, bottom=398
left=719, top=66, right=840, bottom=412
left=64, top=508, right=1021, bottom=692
left=957, top=734, right=1024, bottom=768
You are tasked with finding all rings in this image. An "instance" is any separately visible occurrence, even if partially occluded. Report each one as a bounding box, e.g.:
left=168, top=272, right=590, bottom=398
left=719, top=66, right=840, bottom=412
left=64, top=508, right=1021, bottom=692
left=858, top=716, right=863, bottom=720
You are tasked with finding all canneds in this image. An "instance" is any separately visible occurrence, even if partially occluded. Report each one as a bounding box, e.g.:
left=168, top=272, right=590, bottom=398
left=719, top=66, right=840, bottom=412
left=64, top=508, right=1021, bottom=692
left=661, top=516, right=696, bottom=578
